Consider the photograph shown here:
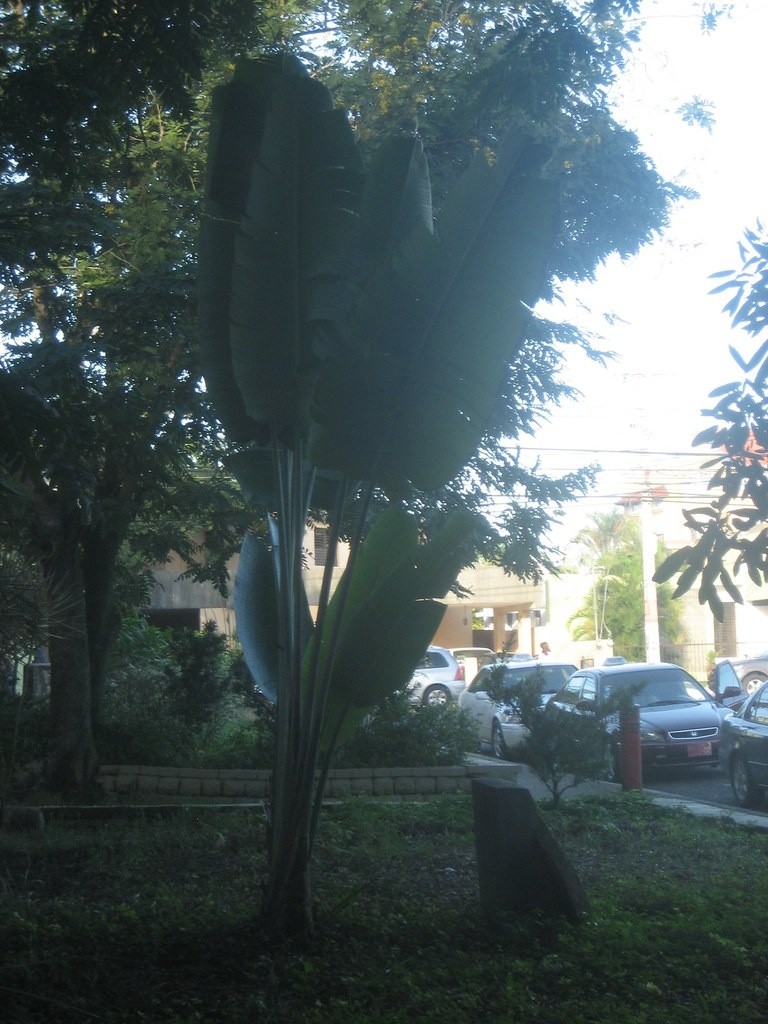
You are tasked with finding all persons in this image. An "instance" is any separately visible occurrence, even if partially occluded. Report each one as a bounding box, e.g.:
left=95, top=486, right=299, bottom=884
left=539, top=641, right=552, bottom=661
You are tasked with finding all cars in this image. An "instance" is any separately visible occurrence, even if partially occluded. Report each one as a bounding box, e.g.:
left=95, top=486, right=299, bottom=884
left=707, top=649, right=768, bottom=698
left=545, top=655, right=751, bottom=784
left=391, top=646, right=467, bottom=710
left=458, top=663, right=595, bottom=760
left=479, top=652, right=535, bottom=671
left=719, top=680, right=768, bottom=809
left=445, top=647, right=494, bottom=689
